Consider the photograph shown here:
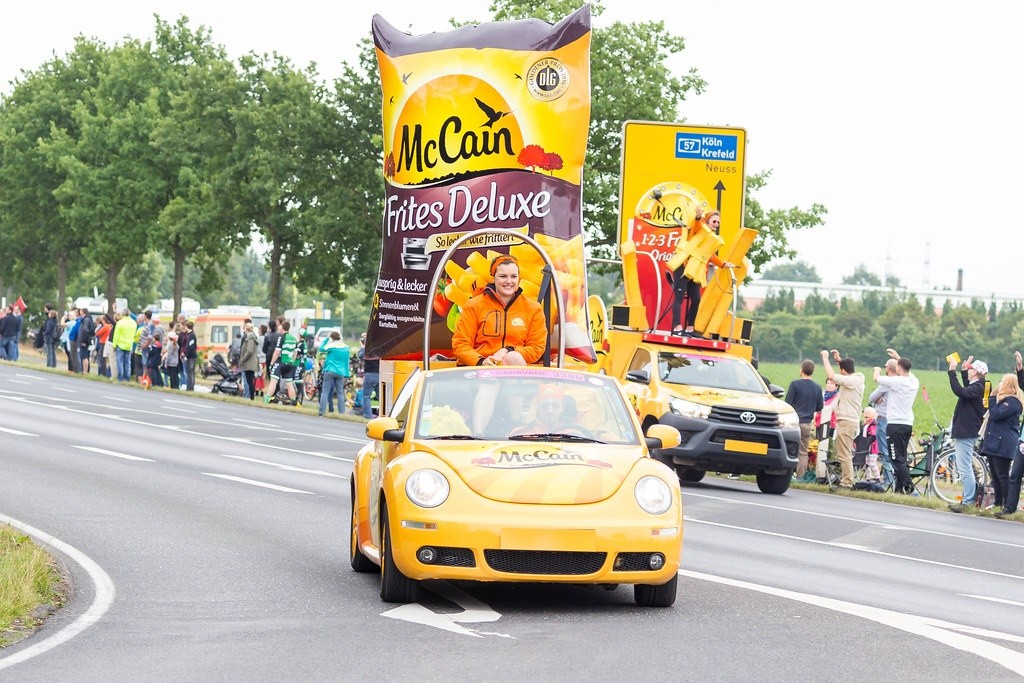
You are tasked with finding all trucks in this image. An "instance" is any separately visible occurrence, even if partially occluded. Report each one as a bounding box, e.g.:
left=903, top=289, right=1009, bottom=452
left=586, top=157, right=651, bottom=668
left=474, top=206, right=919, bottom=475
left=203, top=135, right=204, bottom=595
left=139, top=304, right=344, bottom=379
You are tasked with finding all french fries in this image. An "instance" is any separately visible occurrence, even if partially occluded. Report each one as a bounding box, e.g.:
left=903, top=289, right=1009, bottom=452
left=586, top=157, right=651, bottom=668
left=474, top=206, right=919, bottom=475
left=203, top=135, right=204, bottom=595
left=667, top=194, right=760, bottom=338
left=441, top=232, right=587, bottom=335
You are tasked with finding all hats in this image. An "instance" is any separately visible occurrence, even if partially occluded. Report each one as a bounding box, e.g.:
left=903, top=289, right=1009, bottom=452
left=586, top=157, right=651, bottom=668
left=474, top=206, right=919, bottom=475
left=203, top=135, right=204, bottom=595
left=536, top=385, right=565, bottom=400
left=966, top=360, right=988, bottom=376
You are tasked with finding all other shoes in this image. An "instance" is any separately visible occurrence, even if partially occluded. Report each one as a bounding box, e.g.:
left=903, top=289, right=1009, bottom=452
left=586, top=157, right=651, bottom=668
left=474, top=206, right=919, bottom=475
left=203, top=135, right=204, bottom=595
left=281, top=397, right=297, bottom=407
left=816, top=477, right=826, bottom=484
left=684, top=330, right=704, bottom=339
left=994, top=508, right=1011, bottom=519
left=673, top=329, right=692, bottom=338
left=948, top=503, right=967, bottom=513
left=979, top=504, right=1004, bottom=514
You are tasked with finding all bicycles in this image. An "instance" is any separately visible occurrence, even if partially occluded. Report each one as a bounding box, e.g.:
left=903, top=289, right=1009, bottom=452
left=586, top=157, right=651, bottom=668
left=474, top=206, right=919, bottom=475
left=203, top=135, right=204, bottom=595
left=929, top=421, right=987, bottom=504
left=303, top=350, right=320, bottom=401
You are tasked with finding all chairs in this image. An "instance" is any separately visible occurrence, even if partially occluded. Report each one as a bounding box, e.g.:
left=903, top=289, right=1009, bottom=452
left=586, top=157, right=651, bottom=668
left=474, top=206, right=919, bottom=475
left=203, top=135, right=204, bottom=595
left=906, top=430, right=947, bottom=498
left=822, top=436, right=873, bottom=487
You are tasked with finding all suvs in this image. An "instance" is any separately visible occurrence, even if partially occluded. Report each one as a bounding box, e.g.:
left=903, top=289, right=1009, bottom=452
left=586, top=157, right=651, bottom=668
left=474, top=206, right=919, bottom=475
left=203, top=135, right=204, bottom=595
left=564, top=250, right=802, bottom=496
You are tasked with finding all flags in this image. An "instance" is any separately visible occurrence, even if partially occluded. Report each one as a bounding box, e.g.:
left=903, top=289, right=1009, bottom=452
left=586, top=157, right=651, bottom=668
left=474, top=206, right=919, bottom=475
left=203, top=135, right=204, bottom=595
left=13, top=296, right=27, bottom=312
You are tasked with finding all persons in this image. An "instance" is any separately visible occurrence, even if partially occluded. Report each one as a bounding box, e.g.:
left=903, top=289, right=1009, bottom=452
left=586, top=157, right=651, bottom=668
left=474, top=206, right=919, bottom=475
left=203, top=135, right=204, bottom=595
left=452, top=254, right=548, bottom=369
left=750, top=348, right=1024, bottom=519
left=0, top=298, right=381, bottom=419
left=671, top=205, right=733, bottom=341
left=509, top=385, right=593, bottom=439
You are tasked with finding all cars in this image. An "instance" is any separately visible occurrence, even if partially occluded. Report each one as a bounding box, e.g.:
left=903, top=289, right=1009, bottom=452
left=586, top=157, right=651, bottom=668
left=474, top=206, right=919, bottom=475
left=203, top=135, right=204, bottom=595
left=347, top=365, right=685, bottom=608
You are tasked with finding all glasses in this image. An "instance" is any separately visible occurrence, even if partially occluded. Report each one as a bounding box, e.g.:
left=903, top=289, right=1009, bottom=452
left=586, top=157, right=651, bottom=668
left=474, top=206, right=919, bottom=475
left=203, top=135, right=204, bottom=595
left=708, top=218, right=721, bottom=225
left=883, top=364, right=892, bottom=370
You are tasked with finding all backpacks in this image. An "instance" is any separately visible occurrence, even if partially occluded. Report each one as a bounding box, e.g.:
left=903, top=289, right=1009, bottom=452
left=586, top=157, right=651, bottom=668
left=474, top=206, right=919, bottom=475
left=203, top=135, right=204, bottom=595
left=50, top=320, right=64, bottom=341
left=82, top=315, right=95, bottom=336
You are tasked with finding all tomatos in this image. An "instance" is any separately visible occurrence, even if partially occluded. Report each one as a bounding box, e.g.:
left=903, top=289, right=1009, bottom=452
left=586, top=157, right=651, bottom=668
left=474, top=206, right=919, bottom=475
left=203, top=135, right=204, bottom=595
left=432, top=275, right=454, bottom=317
